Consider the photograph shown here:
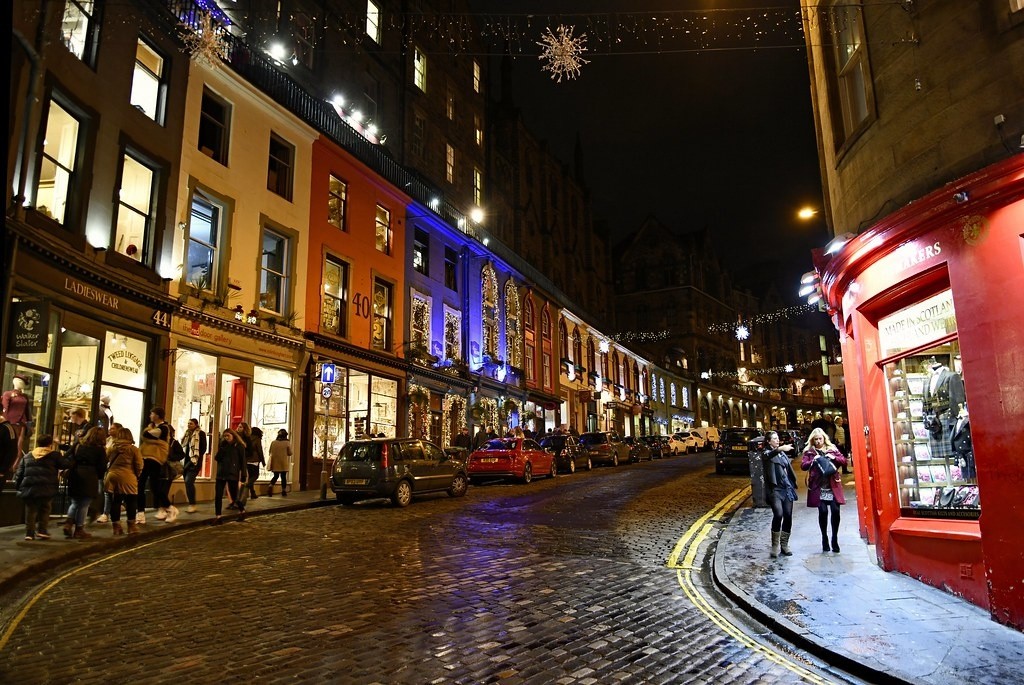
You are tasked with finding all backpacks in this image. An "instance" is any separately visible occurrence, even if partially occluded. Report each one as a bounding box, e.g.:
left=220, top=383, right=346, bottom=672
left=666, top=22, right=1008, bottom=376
left=58, top=468, right=85, bottom=506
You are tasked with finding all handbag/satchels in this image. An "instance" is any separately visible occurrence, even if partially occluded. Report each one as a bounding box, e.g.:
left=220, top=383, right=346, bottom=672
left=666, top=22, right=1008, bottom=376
left=814, top=456, right=837, bottom=476
left=166, top=456, right=183, bottom=483
left=266, top=455, right=272, bottom=471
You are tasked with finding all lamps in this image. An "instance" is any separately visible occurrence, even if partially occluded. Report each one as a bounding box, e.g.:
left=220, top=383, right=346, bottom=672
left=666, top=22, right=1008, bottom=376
left=807, top=291, right=822, bottom=305
left=798, top=279, right=819, bottom=297
left=800, top=270, right=818, bottom=284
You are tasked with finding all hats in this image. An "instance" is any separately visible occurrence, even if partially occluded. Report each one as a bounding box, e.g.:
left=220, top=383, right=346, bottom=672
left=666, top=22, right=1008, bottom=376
left=277, top=429, right=288, bottom=436
left=12, top=373, right=29, bottom=386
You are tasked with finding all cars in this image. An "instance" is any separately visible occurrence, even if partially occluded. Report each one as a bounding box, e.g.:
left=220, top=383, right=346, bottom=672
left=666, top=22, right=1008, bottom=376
left=639, top=435, right=672, bottom=460
left=625, top=436, right=654, bottom=463
left=465, top=437, right=558, bottom=486
left=537, top=435, right=593, bottom=475
left=660, top=434, right=689, bottom=456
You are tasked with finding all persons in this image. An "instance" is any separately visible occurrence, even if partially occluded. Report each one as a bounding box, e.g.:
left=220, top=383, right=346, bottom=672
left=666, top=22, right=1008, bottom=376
left=486, top=426, right=499, bottom=439
left=454, top=427, right=471, bottom=451
left=104, top=407, right=179, bottom=534
left=225, top=421, right=254, bottom=510
left=503, top=424, right=534, bottom=439
left=640, top=430, right=661, bottom=447
left=0, top=413, right=18, bottom=476
left=812, top=413, right=854, bottom=474
left=800, top=427, right=848, bottom=553
left=761, top=430, right=798, bottom=558
left=267, top=428, right=293, bottom=497
left=99, top=391, right=114, bottom=435
left=180, top=418, right=207, bottom=513
left=949, top=409, right=977, bottom=479
left=473, top=425, right=489, bottom=451
left=361, top=432, right=387, bottom=439
left=246, top=427, right=266, bottom=499
left=210, top=427, right=246, bottom=526
left=923, top=362, right=965, bottom=459
left=2, top=373, right=36, bottom=458
left=535, top=425, right=580, bottom=442
left=12, top=407, right=107, bottom=540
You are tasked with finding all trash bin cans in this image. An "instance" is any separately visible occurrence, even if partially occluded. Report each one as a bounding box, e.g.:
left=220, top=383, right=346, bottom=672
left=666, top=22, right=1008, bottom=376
left=444, top=447, right=469, bottom=464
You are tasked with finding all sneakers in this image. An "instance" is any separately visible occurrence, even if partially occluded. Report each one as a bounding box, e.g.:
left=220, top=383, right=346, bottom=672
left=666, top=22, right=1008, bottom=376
left=36, top=528, right=51, bottom=538
left=95, top=503, right=197, bottom=525
left=25, top=529, right=35, bottom=541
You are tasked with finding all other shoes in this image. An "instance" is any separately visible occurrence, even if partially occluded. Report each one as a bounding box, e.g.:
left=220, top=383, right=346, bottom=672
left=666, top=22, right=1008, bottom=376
left=236, top=511, right=248, bottom=521
left=268, top=492, right=272, bottom=497
left=281, top=491, right=288, bottom=497
left=842, top=470, right=852, bottom=474
left=238, top=503, right=244, bottom=511
left=251, top=492, right=259, bottom=499
left=211, top=517, right=224, bottom=526
left=822, top=536, right=830, bottom=551
left=832, top=538, right=840, bottom=551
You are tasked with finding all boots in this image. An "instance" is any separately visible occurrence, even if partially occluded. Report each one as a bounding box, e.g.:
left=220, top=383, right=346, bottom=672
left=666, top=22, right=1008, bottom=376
left=770, top=529, right=781, bottom=558
left=62, top=519, right=141, bottom=539
left=781, top=531, right=793, bottom=556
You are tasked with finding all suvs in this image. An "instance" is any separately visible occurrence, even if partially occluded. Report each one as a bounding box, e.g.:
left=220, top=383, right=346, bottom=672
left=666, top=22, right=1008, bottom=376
left=674, top=429, right=707, bottom=453
left=715, top=427, right=767, bottom=476
left=698, top=427, right=722, bottom=452
left=580, top=430, right=634, bottom=466
left=329, top=436, right=469, bottom=507
left=788, top=427, right=812, bottom=451
left=769, top=430, right=800, bottom=459
